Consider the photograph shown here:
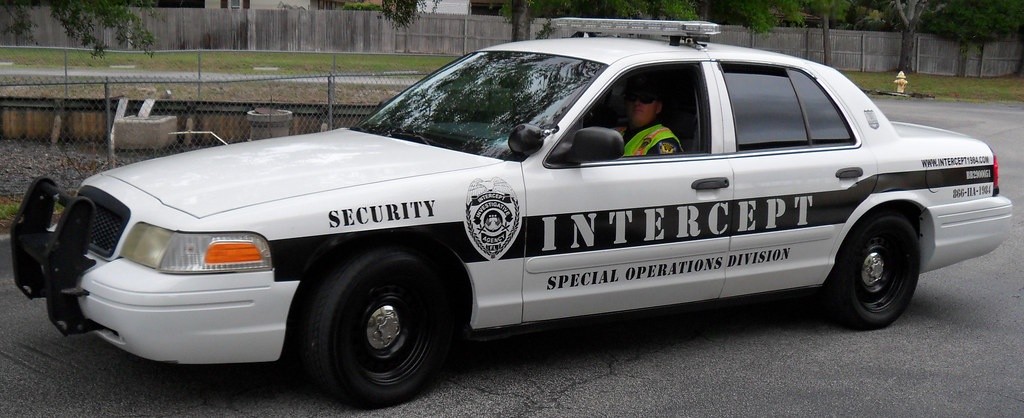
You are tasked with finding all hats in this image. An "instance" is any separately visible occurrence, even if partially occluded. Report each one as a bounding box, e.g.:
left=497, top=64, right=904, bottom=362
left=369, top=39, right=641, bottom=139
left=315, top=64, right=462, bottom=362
left=621, top=73, right=662, bottom=102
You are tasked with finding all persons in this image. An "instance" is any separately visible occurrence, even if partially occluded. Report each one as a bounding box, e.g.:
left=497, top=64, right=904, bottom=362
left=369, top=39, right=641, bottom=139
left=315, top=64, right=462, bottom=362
left=605, top=77, right=683, bottom=157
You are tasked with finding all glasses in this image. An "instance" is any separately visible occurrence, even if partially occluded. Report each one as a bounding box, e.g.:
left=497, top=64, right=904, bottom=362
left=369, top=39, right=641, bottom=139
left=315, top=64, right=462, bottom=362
left=624, top=93, right=659, bottom=104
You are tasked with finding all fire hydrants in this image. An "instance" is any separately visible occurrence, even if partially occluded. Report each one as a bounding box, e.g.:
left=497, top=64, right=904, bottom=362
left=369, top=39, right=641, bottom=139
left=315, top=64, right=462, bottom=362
left=895, top=70, right=909, bottom=93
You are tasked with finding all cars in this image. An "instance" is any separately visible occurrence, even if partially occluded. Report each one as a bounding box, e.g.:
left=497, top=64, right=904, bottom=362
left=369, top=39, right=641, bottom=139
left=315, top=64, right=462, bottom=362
left=9, top=19, right=1014, bottom=412
